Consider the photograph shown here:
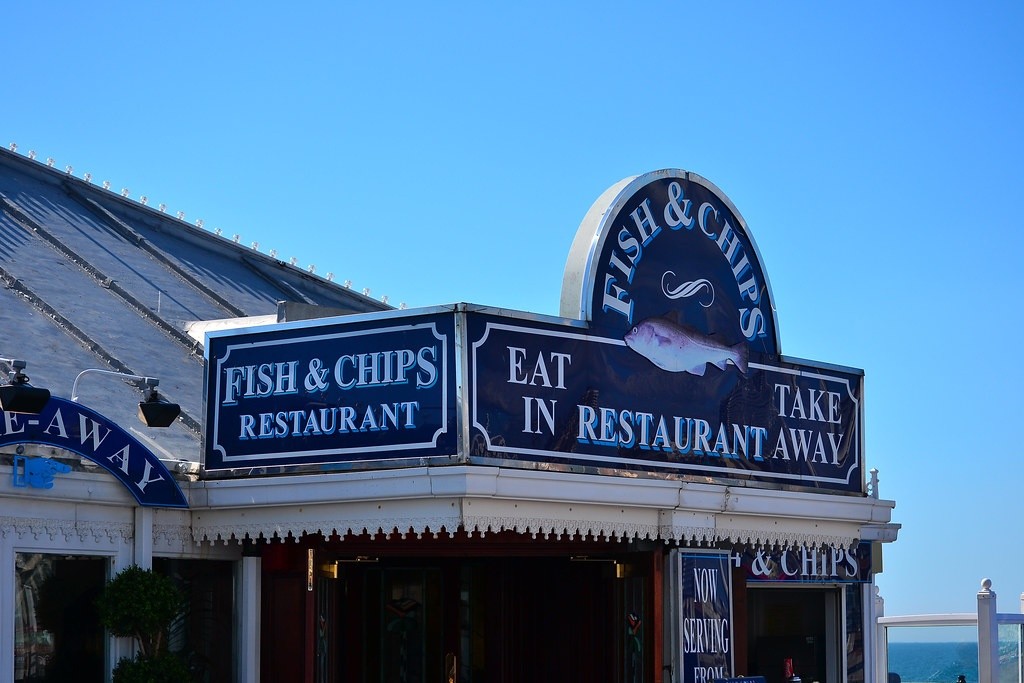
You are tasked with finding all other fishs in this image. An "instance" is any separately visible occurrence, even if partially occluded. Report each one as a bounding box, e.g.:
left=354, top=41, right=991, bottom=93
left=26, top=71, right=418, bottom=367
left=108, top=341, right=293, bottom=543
left=624, top=310, right=750, bottom=376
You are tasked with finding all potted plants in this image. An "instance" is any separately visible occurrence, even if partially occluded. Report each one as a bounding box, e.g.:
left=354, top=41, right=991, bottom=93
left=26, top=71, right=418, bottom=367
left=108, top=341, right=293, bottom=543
left=101, top=563, right=183, bottom=635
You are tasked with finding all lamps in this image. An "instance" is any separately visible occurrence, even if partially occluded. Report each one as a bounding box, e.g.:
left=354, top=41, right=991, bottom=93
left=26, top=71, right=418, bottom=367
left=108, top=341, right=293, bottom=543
left=0, top=357, right=51, bottom=415
left=69, top=368, right=181, bottom=429
